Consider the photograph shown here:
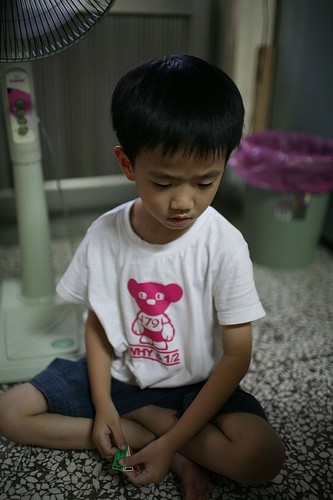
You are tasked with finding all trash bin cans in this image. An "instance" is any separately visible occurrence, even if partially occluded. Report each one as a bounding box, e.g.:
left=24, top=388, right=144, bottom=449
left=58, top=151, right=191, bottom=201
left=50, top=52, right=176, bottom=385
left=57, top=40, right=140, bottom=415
left=231, top=131, right=333, bottom=267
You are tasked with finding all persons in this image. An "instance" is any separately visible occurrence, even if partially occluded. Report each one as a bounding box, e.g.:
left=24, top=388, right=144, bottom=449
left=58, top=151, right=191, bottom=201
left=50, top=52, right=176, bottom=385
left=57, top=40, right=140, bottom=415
left=0, top=54, right=284, bottom=500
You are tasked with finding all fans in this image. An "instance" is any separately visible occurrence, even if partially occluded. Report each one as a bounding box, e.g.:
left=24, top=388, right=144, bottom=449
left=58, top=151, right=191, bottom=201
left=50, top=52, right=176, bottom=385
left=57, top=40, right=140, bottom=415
left=0, top=0, right=117, bottom=386
